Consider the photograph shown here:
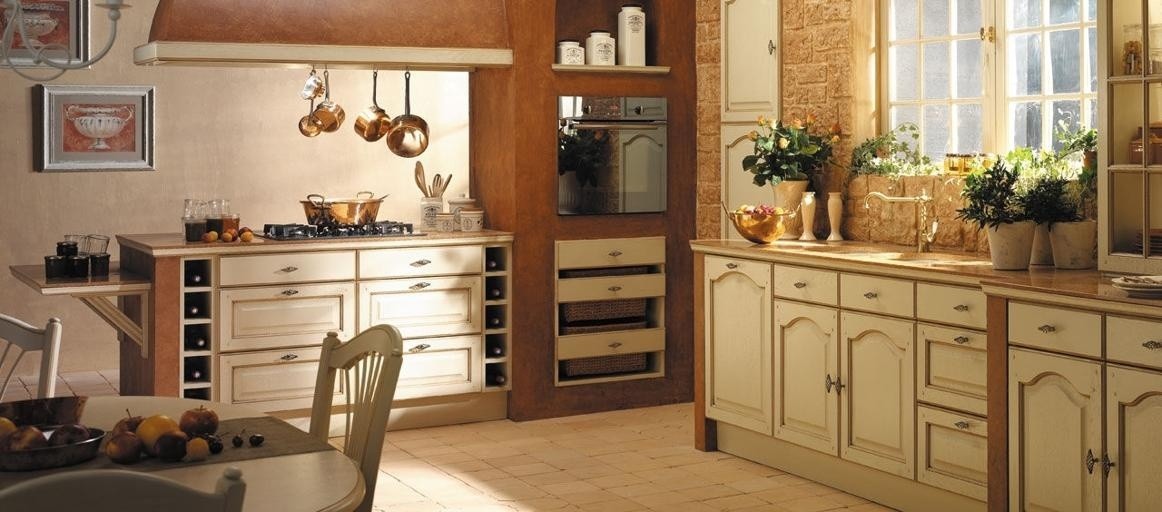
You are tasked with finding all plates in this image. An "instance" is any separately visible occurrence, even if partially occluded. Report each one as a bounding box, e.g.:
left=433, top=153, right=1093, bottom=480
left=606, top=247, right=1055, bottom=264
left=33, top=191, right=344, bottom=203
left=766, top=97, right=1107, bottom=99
left=3, top=421, right=106, bottom=472
left=1110, top=274, right=1162, bottom=299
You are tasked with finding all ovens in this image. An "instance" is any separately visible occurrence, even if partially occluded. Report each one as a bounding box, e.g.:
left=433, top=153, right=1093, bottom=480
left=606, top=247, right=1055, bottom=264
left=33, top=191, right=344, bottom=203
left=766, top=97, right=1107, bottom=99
left=552, top=98, right=670, bottom=216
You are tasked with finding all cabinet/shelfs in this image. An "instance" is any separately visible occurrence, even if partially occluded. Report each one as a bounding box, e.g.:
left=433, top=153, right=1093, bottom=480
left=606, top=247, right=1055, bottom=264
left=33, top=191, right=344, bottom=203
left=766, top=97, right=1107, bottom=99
left=985, top=280, right=1162, bottom=512
left=693, top=240, right=773, bottom=468
left=359, top=250, right=483, bottom=432
left=914, top=274, right=989, bottom=509
left=215, top=247, right=357, bottom=436
left=513, top=0, right=694, bottom=420
left=1095, top=0, right=1162, bottom=272
left=772, top=254, right=912, bottom=510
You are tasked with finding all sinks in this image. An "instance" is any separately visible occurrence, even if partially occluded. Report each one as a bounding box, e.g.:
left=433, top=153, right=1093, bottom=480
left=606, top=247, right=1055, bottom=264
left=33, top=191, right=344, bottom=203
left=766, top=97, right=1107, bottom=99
left=857, top=252, right=980, bottom=263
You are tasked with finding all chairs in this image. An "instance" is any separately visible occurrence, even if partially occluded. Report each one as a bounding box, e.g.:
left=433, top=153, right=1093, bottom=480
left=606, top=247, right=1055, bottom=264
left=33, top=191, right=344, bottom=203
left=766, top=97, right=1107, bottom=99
left=0, top=309, right=64, bottom=398
left=309, top=323, right=403, bottom=512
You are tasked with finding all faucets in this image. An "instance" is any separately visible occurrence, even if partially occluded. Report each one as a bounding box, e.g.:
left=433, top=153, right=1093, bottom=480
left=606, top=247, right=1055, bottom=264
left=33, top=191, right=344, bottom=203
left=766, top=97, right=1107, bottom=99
left=862, top=189, right=939, bottom=252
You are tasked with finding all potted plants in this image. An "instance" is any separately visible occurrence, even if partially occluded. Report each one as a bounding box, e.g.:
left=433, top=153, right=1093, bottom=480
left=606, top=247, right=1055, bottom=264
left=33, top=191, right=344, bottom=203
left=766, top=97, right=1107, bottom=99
left=999, top=129, right=1098, bottom=265
left=1026, top=176, right=1097, bottom=271
left=953, top=152, right=1037, bottom=272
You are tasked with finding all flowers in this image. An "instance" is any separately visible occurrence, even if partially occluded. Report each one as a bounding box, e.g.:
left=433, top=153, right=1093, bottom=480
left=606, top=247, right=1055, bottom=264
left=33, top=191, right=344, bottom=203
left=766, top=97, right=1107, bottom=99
left=560, top=104, right=615, bottom=176
left=740, top=114, right=842, bottom=188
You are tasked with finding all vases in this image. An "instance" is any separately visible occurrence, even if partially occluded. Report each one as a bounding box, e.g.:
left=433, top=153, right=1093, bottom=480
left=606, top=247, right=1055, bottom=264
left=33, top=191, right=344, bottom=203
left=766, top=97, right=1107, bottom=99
left=772, top=180, right=810, bottom=240
left=558, top=170, right=596, bottom=218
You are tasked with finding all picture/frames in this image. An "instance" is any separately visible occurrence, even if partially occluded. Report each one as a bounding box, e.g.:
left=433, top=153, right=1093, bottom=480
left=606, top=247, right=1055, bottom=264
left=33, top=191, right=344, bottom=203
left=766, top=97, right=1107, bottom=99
left=34, top=82, right=157, bottom=171
left=0, top=0, right=91, bottom=70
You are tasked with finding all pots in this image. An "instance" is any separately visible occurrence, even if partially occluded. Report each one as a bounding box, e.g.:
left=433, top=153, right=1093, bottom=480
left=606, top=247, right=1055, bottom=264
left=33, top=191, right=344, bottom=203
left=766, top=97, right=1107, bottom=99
left=298, top=189, right=389, bottom=229
left=353, top=68, right=390, bottom=144
left=384, top=67, right=431, bottom=161
left=296, top=66, right=323, bottom=140
left=311, top=70, right=346, bottom=135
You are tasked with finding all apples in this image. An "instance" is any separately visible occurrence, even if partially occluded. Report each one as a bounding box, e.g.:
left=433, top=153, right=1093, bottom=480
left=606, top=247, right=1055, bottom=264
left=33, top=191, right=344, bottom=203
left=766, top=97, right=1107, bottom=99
left=49, top=425, right=91, bottom=446
left=239, top=228, right=251, bottom=236
left=209, top=231, right=218, bottom=240
left=202, top=233, right=212, bottom=242
left=0, top=416, right=15, bottom=442
left=114, top=414, right=144, bottom=433
left=6, top=423, right=45, bottom=450
left=180, top=408, right=219, bottom=434
left=227, top=229, right=238, bottom=241
left=221, top=234, right=232, bottom=241
left=138, top=415, right=178, bottom=456
left=156, top=433, right=185, bottom=459
left=104, top=433, right=138, bottom=463
left=240, top=231, right=253, bottom=242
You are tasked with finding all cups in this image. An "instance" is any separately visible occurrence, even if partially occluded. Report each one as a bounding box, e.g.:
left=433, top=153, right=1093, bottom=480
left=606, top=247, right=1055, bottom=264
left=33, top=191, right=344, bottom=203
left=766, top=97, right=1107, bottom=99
left=459, top=207, right=486, bottom=232
left=63, top=233, right=89, bottom=256
left=434, top=213, right=456, bottom=233
left=86, top=232, right=111, bottom=256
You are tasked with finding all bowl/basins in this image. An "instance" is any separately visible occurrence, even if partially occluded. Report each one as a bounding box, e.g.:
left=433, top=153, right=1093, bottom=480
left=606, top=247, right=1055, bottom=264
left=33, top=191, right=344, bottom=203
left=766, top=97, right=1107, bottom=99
left=715, top=198, right=804, bottom=244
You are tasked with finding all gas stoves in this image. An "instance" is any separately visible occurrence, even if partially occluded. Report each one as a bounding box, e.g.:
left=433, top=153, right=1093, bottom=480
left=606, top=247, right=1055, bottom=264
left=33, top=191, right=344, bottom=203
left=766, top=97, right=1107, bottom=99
left=255, top=217, right=433, bottom=239
